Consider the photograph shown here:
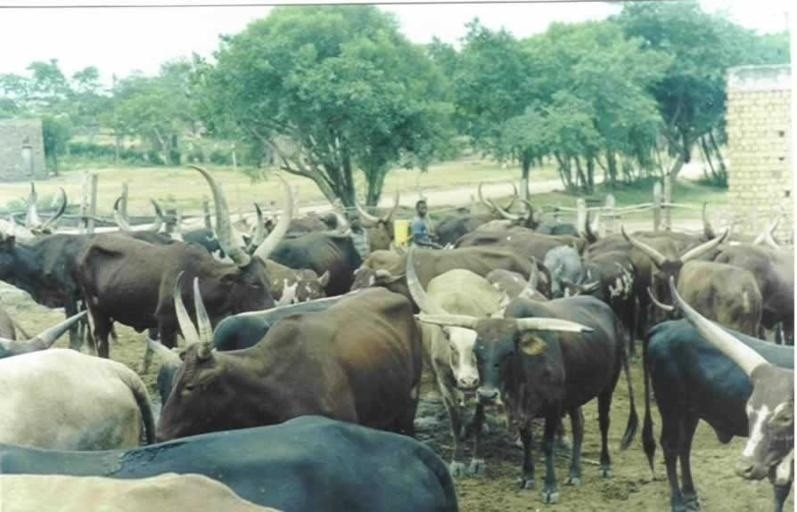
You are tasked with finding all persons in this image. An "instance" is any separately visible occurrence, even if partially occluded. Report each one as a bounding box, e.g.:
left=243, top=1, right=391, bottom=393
left=410, top=200, right=443, bottom=249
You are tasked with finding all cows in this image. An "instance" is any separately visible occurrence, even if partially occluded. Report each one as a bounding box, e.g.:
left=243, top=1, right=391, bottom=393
left=1, top=162, right=794, bottom=512
